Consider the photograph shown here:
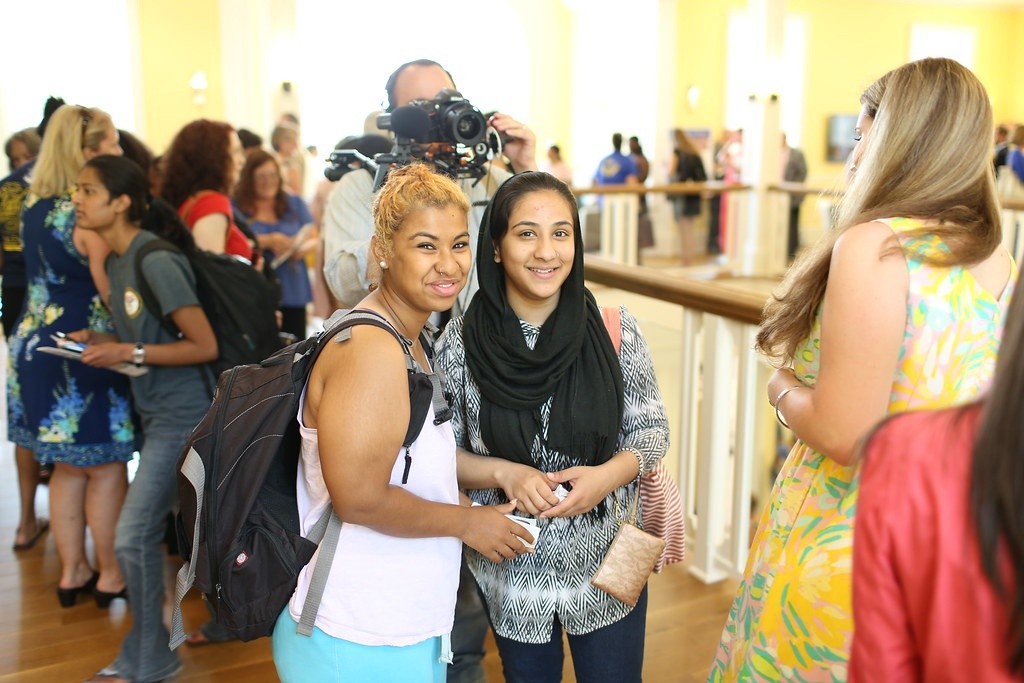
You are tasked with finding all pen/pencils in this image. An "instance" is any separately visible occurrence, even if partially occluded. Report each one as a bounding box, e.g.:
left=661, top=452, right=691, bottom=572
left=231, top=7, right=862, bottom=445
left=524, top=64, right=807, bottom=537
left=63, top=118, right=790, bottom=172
left=56, top=331, right=77, bottom=343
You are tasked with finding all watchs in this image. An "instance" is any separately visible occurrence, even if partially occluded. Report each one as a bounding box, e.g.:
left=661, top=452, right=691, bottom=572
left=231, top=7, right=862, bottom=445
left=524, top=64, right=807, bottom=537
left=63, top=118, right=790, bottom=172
left=133, top=342, right=145, bottom=366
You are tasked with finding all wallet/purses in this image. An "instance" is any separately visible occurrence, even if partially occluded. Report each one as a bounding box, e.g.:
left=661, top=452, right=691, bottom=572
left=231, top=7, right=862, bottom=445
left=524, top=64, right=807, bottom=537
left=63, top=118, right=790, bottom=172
left=589, top=523, right=666, bottom=609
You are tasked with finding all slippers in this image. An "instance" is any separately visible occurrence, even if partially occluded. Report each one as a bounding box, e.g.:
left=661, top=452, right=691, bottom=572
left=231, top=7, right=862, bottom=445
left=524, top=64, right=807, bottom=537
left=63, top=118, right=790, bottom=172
left=93, top=584, right=128, bottom=610
left=58, top=570, right=101, bottom=608
left=14, top=518, right=51, bottom=549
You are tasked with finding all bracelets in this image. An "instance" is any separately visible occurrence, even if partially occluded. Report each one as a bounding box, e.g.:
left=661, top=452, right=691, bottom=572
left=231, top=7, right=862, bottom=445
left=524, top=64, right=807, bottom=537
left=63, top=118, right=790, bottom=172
left=774, top=386, right=800, bottom=429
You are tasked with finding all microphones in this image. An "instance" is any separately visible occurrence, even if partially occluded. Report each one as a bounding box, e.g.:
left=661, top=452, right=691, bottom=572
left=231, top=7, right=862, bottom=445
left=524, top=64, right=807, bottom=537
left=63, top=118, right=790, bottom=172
left=377, top=106, right=430, bottom=138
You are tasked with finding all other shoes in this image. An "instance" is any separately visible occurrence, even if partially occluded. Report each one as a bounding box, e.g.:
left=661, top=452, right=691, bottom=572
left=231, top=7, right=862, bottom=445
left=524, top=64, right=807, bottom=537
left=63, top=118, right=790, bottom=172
left=88, top=658, right=181, bottom=682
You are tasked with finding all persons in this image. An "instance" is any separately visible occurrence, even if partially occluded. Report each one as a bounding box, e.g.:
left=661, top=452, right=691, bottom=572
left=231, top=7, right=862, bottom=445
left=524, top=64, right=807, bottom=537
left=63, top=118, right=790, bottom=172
left=435, top=172, right=672, bottom=683
left=267, top=165, right=534, bottom=683
left=325, top=58, right=536, bottom=683
left=0, top=96, right=392, bottom=683
left=628, top=136, right=655, bottom=265
left=992, top=124, right=1024, bottom=184
left=593, top=131, right=639, bottom=213
left=843, top=251, right=1024, bottom=683
left=547, top=146, right=572, bottom=184
left=667, top=124, right=810, bottom=271
left=705, top=57, right=1024, bottom=683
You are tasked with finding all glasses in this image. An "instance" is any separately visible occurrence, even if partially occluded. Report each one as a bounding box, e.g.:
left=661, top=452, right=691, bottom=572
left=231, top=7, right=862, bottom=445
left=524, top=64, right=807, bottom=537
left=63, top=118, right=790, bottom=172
left=75, top=104, right=93, bottom=154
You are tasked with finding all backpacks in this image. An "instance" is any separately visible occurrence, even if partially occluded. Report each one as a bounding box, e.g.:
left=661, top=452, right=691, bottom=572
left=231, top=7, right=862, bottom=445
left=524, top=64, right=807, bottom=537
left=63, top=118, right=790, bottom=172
left=165, top=308, right=451, bottom=652
left=135, top=239, right=284, bottom=399
